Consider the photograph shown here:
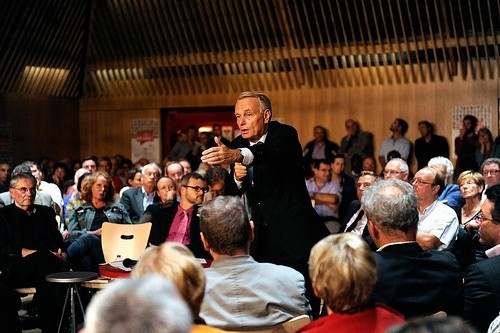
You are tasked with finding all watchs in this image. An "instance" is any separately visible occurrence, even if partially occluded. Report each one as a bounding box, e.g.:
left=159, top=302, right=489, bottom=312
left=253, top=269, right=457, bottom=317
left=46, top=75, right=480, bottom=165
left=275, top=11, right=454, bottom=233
left=237, top=149, right=245, bottom=163
left=310, top=192, right=316, bottom=199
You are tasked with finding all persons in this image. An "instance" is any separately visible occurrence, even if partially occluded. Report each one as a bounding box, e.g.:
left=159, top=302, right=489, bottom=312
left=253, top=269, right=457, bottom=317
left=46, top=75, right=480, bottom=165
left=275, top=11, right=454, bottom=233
left=80, top=273, right=193, bottom=333
left=377, top=118, right=413, bottom=173
left=62, top=171, right=132, bottom=272
left=201, top=90, right=332, bottom=313
left=194, top=193, right=307, bottom=329
left=138, top=172, right=211, bottom=259
left=0, top=115, right=500, bottom=333
left=461, top=182, right=500, bottom=333
left=359, top=178, right=464, bottom=321
left=413, top=121, right=450, bottom=173
left=340, top=117, right=374, bottom=174
left=294, top=232, right=409, bottom=333
left=0, top=172, right=68, bottom=333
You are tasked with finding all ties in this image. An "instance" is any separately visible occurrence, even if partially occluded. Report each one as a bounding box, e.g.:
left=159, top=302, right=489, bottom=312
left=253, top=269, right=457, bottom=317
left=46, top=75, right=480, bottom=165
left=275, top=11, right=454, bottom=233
left=345, top=210, right=365, bottom=233
left=174, top=210, right=188, bottom=244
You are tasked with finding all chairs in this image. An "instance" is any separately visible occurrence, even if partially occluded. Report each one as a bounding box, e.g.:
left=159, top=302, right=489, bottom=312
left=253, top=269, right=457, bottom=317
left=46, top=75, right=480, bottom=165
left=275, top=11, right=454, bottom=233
left=16, top=222, right=447, bottom=333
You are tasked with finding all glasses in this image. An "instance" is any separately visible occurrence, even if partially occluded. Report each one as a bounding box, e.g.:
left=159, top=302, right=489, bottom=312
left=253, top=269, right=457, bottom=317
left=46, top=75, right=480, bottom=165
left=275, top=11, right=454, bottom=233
left=182, top=184, right=209, bottom=194
left=479, top=214, right=493, bottom=222
left=12, top=186, right=36, bottom=193
left=383, top=170, right=406, bottom=175
left=411, top=179, right=433, bottom=186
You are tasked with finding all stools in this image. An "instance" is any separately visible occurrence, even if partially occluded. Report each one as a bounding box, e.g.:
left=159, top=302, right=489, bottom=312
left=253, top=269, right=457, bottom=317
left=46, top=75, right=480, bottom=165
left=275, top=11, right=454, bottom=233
left=46, top=272, right=98, bottom=333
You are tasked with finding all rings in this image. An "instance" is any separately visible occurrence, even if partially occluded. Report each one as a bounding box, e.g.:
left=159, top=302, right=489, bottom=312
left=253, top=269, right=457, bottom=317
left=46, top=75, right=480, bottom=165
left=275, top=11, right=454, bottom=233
left=213, top=156, right=219, bottom=160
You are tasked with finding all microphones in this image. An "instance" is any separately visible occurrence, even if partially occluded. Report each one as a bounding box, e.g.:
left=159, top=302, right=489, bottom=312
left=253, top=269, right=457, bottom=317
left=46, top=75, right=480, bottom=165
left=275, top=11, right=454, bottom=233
left=123, top=258, right=138, bottom=268
